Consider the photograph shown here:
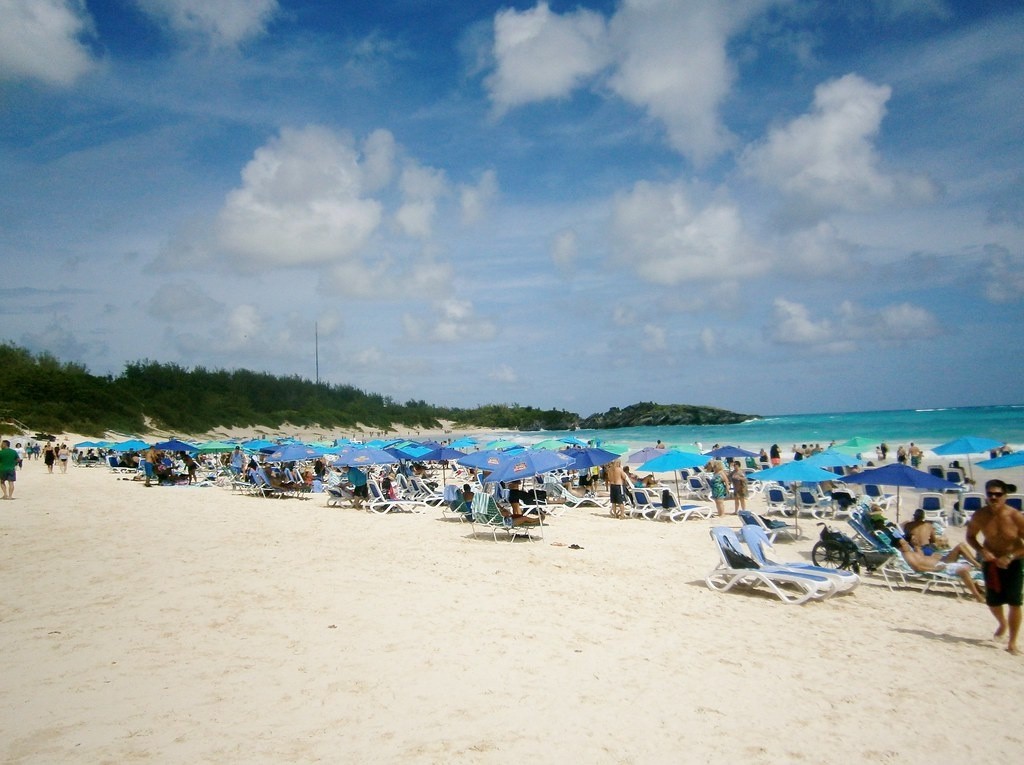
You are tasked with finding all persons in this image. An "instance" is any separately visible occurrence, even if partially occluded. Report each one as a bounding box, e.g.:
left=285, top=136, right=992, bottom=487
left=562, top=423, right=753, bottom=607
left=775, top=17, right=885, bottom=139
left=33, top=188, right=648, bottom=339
left=891, top=537, right=987, bottom=604
left=178, top=450, right=199, bottom=485
left=463, top=484, right=475, bottom=500
left=144, top=445, right=157, bottom=487
left=14, top=442, right=25, bottom=471
left=770, top=443, right=782, bottom=467
left=43, top=440, right=57, bottom=474
left=607, top=459, right=632, bottom=519
left=909, top=442, right=921, bottom=469
left=497, top=502, right=545, bottom=526
left=709, top=460, right=730, bottom=518
left=728, top=460, right=748, bottom=516
left=0, top=439, right=23, bottom=501
left=896, top=446, right=908, bottom=465
left=508, top=480, right=521, bottom=514
left=228, top=445, right=246, bottom=486
left=57, top=444, right=71, bottom=474
left=907, top=534, right=982, bottom=569
left=905, top=509, right=936, bottom=546
left=341, top=465, right=370, bottom=511
left=965, top=479, right=1024, bottom=658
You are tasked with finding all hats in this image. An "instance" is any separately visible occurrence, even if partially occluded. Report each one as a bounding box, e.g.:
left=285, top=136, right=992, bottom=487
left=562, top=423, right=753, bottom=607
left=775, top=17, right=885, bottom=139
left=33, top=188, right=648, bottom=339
left=235, top=446, right=240, bottom=450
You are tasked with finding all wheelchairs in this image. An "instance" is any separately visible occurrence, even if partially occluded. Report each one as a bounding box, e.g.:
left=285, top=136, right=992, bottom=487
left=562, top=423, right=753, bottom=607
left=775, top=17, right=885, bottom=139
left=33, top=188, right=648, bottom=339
left=812, top=522, right=877, bottom=574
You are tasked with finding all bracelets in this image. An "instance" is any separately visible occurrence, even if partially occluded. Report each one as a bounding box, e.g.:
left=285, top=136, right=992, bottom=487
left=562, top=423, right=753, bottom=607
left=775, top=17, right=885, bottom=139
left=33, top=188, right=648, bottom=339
left=1008, top=552, right=1014, bottom=560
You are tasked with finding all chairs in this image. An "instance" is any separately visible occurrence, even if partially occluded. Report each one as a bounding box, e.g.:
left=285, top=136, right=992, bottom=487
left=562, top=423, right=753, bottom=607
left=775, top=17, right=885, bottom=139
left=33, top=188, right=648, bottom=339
left=706, top=511, right=859, bottom=608
left=71, top=454, right=610, bottom=545
left=762, top=462, right=1024, bottom=602
left=612, top=460, right=771, bottom=521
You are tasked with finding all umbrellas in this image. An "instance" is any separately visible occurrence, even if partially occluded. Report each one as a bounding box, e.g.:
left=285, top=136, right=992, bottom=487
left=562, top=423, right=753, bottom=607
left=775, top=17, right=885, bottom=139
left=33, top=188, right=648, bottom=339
left=74, top=432, right=1024, bottom=544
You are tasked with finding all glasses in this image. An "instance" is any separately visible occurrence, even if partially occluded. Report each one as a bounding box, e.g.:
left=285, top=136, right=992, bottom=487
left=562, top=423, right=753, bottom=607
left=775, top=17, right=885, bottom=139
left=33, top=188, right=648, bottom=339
left=985, top=491, right=1005, bottom=497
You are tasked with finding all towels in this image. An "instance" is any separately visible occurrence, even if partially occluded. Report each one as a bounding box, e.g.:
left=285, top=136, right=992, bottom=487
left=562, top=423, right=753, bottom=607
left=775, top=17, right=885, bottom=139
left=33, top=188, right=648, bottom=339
left=443, top=484, right=461, bottom=501
left=470, top=491, right=490, bottom=519
